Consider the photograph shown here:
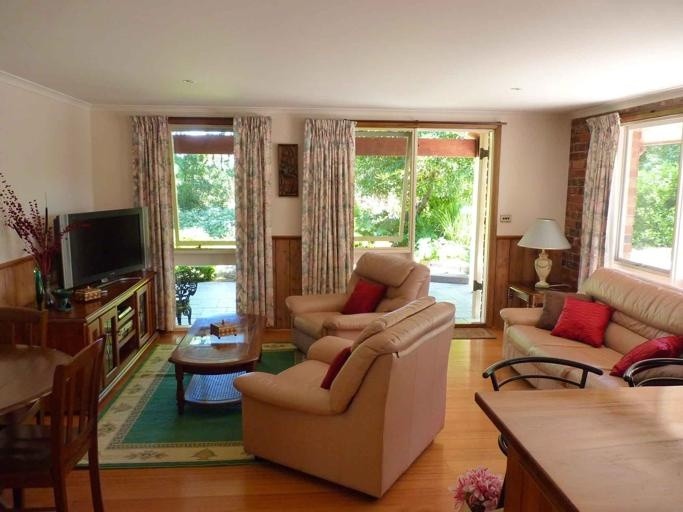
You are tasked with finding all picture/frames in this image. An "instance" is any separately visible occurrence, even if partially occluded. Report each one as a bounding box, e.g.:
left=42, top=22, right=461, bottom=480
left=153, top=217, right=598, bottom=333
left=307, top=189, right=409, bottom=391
left=277, top=143, right=299, bottom=197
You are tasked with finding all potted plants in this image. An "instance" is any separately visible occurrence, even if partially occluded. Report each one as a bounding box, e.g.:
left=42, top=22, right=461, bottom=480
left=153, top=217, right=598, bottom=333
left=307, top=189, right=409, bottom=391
left=173, top=243, right=218, bottom=325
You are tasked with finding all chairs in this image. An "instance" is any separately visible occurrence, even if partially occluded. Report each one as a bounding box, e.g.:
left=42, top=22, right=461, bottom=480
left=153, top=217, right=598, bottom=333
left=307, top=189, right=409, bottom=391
left=0, top=304, right=48, bottom=423
left=481, top=356, right=603, bottom=507
left=621, top=356, right=682, bottom=386
left=0, top=336, right=108, bottom=511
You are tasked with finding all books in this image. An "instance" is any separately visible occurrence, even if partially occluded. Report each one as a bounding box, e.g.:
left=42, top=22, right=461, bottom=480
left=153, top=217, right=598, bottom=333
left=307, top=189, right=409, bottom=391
left=116, top=305, right=132, bottom=319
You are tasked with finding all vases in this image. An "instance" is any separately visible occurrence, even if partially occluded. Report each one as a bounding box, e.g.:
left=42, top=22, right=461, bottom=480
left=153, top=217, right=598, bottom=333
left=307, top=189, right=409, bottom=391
left=42, top=273, right=55, bottom=310
left=33, top=269, right=44, bottom=310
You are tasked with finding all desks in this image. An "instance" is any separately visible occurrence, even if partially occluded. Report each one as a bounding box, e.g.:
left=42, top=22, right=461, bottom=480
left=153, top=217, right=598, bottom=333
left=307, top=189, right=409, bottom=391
left=0, top=342, right=77, bottom=509
left=473, top=388, right=682, bottom=512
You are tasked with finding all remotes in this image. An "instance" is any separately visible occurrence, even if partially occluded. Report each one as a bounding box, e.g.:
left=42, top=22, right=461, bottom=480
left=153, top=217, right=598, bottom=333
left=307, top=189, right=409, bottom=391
left=51, top=290, right=74, bottom=295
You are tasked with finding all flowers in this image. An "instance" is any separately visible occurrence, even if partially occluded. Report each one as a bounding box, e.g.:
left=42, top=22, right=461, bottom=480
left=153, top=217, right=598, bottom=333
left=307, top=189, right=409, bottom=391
left=0, top=171, right=72, bottom=275
left=447, top=465, right=503, bottom=512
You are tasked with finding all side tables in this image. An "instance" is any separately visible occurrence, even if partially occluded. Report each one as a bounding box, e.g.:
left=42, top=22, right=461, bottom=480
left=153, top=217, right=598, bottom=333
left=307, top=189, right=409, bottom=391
left=505, top=279, right=577, bottom=308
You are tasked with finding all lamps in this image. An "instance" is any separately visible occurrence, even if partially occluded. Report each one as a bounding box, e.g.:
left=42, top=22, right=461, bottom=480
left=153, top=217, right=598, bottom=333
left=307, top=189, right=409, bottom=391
left=516, top=219, right=571, bottom=288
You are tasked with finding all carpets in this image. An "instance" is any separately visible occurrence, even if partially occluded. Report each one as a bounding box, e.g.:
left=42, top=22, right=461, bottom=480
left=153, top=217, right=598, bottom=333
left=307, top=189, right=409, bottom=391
left=74, top=340, right=305, bottom=469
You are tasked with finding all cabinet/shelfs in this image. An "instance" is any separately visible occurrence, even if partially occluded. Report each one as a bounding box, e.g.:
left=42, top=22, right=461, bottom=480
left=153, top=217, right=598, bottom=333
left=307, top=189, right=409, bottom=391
left=21, top=268, right=160, bottom=413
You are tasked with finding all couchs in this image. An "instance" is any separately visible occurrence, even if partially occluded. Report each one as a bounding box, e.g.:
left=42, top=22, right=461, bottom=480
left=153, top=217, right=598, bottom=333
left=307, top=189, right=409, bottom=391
left=231, top=295, right=456, bottom=497
left=497, top=267, right=682, bottom=388
left=283, top=251, right=430, bottom=355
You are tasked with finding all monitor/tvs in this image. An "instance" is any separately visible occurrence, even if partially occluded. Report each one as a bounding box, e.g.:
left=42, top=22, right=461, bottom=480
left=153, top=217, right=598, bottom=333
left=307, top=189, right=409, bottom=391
left=53, top=206, right=152, bottom=290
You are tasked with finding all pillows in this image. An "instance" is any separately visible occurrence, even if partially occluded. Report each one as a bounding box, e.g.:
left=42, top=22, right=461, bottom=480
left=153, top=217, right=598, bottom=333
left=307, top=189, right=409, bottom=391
left=550, top=296, right=614, bottom=349
left=343, top=280, right=388, bottom=313
left=319, top=345, right=351, bottom=389
left=608, top=333, right=683, bottom=377
left=536, top=290, right=594, bottom=330
